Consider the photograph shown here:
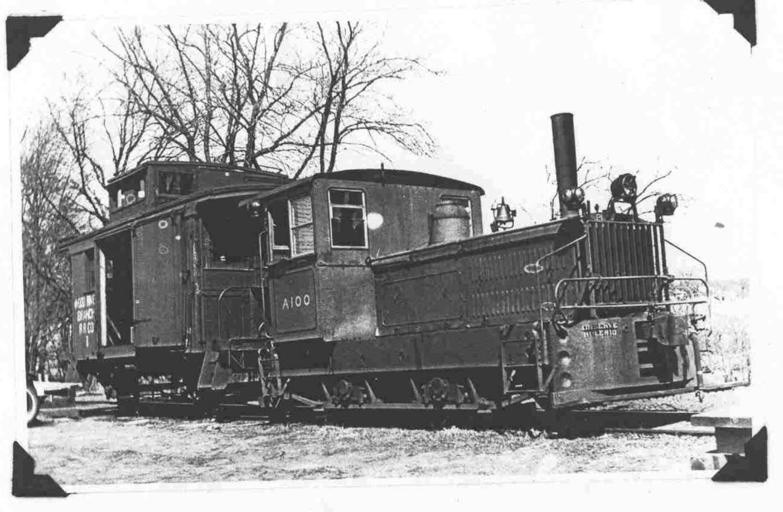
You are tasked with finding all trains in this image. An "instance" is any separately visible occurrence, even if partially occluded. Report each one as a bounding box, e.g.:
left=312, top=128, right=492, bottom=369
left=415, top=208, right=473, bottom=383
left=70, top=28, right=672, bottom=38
left=69, top=100, right=707, bottom=431
